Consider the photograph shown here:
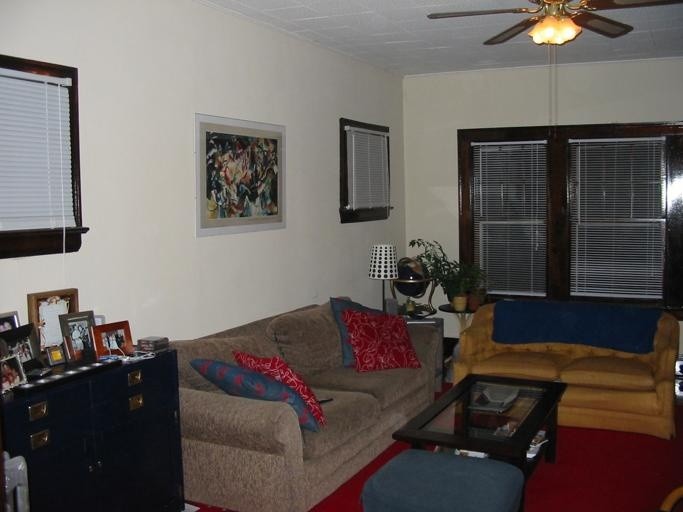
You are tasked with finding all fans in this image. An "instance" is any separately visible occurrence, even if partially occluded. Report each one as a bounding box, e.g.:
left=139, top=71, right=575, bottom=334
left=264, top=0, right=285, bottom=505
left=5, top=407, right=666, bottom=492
left=426, top=1, right=682, bottom=46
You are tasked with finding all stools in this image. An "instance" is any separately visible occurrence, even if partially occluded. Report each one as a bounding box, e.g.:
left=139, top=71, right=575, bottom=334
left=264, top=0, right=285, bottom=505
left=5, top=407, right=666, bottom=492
left=360, top=449, right=527, bottom=511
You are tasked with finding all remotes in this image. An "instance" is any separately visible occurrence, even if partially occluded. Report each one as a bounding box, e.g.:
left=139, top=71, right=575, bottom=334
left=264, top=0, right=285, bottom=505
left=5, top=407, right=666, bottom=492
left=318, top=397, right=333, bottom=403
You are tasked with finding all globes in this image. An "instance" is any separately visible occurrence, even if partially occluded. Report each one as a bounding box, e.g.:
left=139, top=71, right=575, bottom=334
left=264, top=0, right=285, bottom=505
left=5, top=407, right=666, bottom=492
left=393, top=257, right=433, bottom=310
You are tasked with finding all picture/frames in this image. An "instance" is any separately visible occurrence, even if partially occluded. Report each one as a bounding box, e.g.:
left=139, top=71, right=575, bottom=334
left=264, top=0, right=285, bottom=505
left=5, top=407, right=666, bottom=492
left=194, top=111, right=289, bottom=236
left=1, top=289, right=135, bottom=393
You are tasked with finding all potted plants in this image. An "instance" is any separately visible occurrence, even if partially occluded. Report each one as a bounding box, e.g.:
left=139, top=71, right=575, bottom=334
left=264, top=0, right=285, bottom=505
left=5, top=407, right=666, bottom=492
left=409, top=236, right=487, bottom=311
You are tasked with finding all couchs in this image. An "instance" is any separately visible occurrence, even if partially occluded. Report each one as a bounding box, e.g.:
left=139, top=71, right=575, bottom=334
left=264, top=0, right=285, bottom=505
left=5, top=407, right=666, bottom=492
left=451, top=297, right=680, bottom=441
left=168, top=304, right=444, bottom=512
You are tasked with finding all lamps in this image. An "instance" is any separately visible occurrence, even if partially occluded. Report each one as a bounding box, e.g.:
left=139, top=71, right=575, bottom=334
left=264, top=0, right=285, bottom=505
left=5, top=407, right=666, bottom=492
left=519, top=0, right=597, bottom=46
left=368, top=243, right=399, bottom=313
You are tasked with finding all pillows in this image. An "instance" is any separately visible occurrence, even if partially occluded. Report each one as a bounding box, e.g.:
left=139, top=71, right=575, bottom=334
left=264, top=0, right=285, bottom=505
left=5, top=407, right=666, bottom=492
left=165, top=297, right=422, bottom=434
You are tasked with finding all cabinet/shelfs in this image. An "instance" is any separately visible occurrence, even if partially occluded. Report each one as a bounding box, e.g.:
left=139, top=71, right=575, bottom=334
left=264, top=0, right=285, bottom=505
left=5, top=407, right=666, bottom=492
left=0, top=347, right=185, bottom=512
